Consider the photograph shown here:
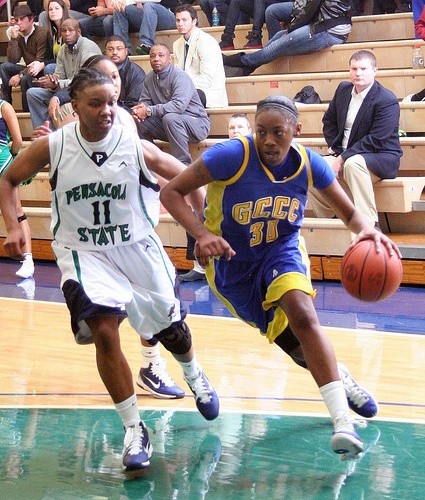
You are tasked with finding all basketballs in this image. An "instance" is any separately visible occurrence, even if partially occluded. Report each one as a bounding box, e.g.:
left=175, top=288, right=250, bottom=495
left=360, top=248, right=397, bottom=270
left=341, top=238, right=404, bottom=302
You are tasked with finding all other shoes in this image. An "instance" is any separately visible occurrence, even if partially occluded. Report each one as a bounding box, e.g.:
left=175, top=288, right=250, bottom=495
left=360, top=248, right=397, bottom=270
left=178, top=269, right=207, bottom=282
left=135, top=43, right=151, bottom=54
left=219, top=39, right=234, bottom=51
left=221, top=52, right=258, bottom=75
left=15, top=261, right=35, bottom=278
left=242, top=38, right=263, bottom=49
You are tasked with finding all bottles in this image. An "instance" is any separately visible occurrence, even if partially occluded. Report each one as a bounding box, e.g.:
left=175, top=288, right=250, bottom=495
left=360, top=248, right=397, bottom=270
left=411, top=45, right=424, bottom=70
left=212, top=5, right=220, bottom=26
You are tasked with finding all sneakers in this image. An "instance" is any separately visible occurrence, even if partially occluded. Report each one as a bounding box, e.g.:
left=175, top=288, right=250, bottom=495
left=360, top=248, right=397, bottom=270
left=331, top=416, right=365, bottom=461
left=135, top=358, right=185, bottom=398
left=119, top=419, right=152, bottom=472
left=335, top=363, right=378, bottom=418
left=183, top=364, right=219, bottom=421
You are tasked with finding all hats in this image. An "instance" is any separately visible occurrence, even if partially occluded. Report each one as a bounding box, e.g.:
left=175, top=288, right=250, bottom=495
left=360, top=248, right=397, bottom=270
left=13, top=5, right=35, bottom=19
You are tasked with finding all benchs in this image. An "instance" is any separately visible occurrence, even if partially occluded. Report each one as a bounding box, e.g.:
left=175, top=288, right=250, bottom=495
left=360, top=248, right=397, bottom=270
left=0, top=0, right=425, bottom=288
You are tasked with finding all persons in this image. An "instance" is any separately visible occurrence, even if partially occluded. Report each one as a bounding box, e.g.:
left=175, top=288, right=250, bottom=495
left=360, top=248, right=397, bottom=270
left=159, top=94, right=401, bottom=455
left=217, top=0, right=353, bottom=77
left=31, top=54, right=184, bottom=400
left=178, top=114, right=252, bottom=282
left=309, top=50, right=404, bottom=230
left=0, top=99, right=35, bottom=278
left=373, top=0, right=425, bottom=39
left=0, top=0, right=229, bottom=166
left=0, top=67, right=220, bottom=472
